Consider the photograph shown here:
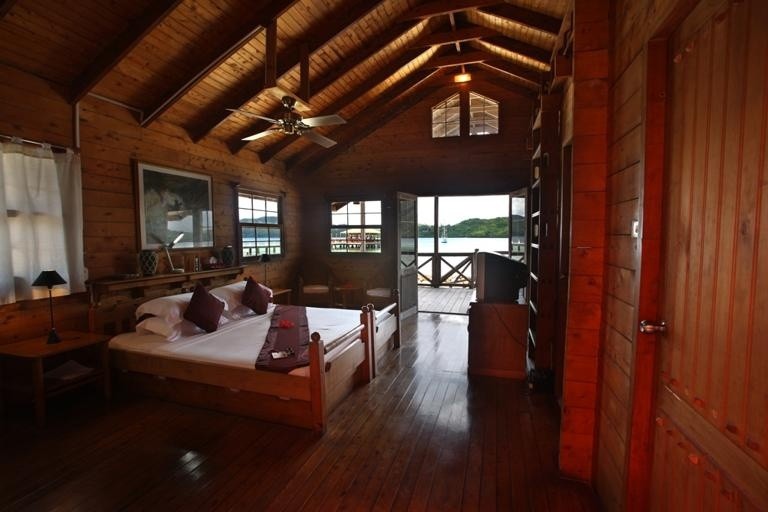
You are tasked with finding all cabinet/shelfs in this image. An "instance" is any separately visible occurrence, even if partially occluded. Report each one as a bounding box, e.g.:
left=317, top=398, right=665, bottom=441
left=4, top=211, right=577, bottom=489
left=528, top=95, right=562, bottom=371
left=468, top=295, right=528, bottom=380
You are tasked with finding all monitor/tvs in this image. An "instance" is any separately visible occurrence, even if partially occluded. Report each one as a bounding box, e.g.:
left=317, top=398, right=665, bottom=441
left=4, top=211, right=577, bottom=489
left=478, top=249, right=529, bottom=303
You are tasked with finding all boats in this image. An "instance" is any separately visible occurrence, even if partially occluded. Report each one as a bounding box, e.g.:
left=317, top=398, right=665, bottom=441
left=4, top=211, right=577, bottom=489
left=441, top=237, right=447, bottom=244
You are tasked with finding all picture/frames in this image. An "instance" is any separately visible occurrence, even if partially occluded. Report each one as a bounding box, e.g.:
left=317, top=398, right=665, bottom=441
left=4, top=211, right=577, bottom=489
left=131, top=158, right=215, bottom=253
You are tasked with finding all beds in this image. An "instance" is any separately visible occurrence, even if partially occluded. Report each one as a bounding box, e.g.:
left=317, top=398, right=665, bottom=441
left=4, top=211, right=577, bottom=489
left=86, top=266, right=401, bottom=436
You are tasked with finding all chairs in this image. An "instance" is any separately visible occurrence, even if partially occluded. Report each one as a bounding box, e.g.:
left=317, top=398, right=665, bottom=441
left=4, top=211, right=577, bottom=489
left=297, top=262, right=336, bottom=308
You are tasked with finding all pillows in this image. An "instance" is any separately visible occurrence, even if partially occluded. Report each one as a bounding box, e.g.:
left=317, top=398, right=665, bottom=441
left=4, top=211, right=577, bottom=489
left=209, top=281, right=274, bottom=311
left=135, top=292, right=194, bottom=320
left=242, top=276, right=270, bottom=314
left=136, top=316, right=228, bottom=342
left=184, top=283, right=225, bottom=333
left=222, top=304, right=273, bottom=320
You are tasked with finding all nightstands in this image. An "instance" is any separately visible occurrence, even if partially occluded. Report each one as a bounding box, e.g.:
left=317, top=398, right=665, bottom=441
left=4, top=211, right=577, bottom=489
left=0, top=331, right=112, bottom=433
left=272, top=287, right=292, bottom=305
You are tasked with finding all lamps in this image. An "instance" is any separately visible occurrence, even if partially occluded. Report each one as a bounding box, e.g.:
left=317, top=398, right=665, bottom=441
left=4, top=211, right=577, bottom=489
left=258, top=254, right=273, bottom=289
left=31, top=271, right=67, bottom=344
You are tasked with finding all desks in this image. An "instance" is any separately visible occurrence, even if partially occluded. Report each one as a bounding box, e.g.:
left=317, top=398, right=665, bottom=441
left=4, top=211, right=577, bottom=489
left=333, top=286, right=364, bottom=308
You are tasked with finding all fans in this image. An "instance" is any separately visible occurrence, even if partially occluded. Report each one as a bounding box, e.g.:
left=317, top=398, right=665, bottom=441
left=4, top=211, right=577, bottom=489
left=226, top=96, right=347, bottom=149
